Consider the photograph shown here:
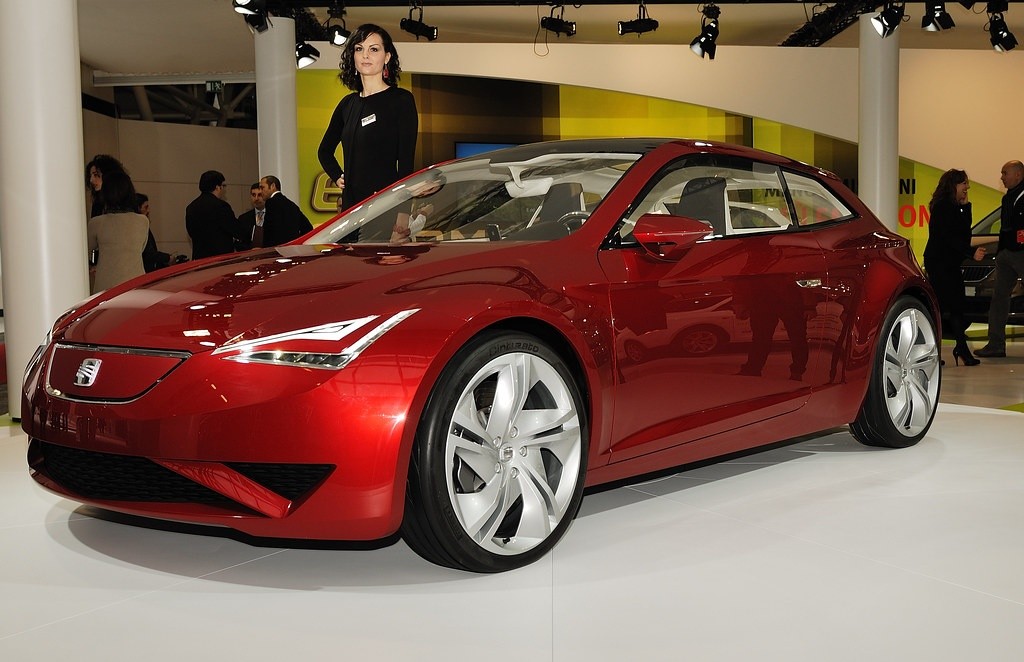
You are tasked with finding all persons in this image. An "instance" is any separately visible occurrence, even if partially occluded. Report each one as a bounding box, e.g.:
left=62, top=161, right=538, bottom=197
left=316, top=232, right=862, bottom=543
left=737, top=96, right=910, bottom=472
left=85, top=154, right=135, bottom=276
left=133, top=193, right=178, bottom=274
left=233, top=183, right=265, bottom=252
left=259, top=175, right=313, bottom=248
left=923, top=167, right=988, bottom=366
left=186, top=170, right=238, bottom=260
left=973, top=159, right=1024, bottom=357
left=733, top=233, right=818, bottom=381
left=359, top=169, right=447, bottom=243
left=318, top=23, right=418, bottom=243
left=87, top=172, right=150, bottom=296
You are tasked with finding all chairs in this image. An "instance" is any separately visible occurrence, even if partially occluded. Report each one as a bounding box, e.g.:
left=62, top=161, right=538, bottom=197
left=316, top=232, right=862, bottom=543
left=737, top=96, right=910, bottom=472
left=675, top=176, right=734, bottom=238
left=540, top=182, right=587, bottom=230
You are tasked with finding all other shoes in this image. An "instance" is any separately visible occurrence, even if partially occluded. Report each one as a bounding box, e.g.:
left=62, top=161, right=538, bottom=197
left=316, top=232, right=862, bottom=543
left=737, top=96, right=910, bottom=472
left=789, top=363, right=803, bottom=381
left=738, top=365, right=761, bottom=376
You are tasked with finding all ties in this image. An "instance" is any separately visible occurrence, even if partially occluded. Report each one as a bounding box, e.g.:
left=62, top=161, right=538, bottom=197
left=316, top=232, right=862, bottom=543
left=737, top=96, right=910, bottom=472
left=256, top=212, right=263, bottom=226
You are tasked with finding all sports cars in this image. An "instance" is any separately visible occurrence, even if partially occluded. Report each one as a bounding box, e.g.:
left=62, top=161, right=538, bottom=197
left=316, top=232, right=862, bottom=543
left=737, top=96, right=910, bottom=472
left=18, top=131, right=945, bottom=577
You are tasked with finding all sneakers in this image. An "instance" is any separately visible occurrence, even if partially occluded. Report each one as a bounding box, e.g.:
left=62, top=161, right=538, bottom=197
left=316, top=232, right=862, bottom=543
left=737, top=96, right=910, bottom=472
left=975, top=342, right=1006, bottom=357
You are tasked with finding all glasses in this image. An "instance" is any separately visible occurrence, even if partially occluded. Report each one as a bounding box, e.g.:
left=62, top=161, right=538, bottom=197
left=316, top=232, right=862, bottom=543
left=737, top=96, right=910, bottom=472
left=220, top=184, right=227, bottom=189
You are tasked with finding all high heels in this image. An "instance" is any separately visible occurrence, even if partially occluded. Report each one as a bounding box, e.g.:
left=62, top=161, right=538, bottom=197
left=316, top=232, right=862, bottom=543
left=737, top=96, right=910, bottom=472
left=952, top=345, right=981, bottom=366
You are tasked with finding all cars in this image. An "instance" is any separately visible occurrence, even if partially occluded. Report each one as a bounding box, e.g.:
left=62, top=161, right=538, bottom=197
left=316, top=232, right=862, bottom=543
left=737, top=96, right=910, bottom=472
left=959, top=203, right=1024, bottom=314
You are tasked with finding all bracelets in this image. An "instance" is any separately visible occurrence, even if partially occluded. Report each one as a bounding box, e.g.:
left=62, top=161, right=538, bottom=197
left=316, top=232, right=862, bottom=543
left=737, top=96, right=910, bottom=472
left=393, top=224, right=411, bottom=236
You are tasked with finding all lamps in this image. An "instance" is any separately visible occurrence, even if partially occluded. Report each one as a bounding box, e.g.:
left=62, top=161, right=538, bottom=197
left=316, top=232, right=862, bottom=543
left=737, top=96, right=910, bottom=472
left=618, top=5, right=659, bottom=37
left=322, top=0, right=351, bottom=46
left=922, top=2, right=956, bottom=31
left=871, top=0, right=910, bottom=38
left=400, top=0, right=438, bottom=41
left=295, top=33, right=320, bottom=69
left=690, top=2, right=721, bottom=60
left=232, top=0, right=274, bottom=33
left=535, top=4, right=576, bottom=37
left=984, top=1, right=1018, bottom=53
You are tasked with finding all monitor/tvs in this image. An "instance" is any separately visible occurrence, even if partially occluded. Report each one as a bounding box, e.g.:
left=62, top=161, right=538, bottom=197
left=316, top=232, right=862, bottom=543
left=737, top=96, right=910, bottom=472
left=454, top=142, right=517, bottom=160
